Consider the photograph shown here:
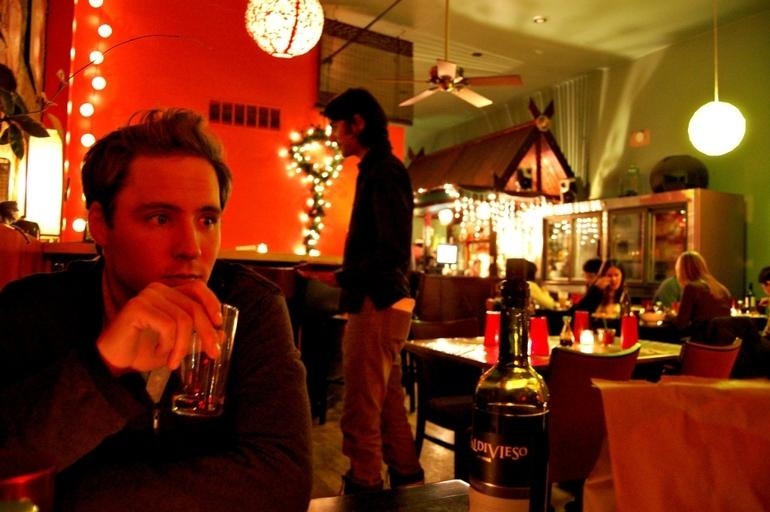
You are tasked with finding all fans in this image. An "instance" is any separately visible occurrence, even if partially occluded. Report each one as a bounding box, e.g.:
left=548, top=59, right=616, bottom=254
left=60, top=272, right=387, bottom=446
left=376, top=1, right=524, bottom=108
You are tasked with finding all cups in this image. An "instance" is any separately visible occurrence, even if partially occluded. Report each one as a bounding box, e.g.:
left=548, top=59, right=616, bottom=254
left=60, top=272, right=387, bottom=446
left=169, top=302, right=242, bottom=417
left=475, top=265, right=759, bottom=366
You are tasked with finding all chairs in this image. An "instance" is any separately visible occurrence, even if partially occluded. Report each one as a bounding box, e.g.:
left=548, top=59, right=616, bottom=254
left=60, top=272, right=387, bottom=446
left=319, top=269, right=424, bottom=424
left=680, top=336, right=742, bottom=378
left=411, top=317, right=481, bottom=459
left=583, top=378, right=770, bottom=511
left=242, top=261, right=309, bottom=358
left=544, top=343, right=641, bottom=511
left=707, top=316, right=769, bottom=338
left=418, top=275, right=491, bottom=321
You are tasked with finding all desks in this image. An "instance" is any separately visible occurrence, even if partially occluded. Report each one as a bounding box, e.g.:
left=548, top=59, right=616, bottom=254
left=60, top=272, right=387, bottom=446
left=40, top=242, right=344, bottom=348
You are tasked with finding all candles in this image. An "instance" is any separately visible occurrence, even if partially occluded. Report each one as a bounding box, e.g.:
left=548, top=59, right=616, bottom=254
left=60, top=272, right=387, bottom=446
left=573, top=311, right=589, bottom=343
left=485, top=311, right=502, bottom=347
left=621, top=316, right=638, bottom=349
left=529, top=317, right=549, bottom=356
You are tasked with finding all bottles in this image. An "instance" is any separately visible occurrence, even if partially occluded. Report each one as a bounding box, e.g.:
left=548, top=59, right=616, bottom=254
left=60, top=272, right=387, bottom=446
left=466, top=252, right=555, bottom=512
left=623, top=162, right=641, bottom=195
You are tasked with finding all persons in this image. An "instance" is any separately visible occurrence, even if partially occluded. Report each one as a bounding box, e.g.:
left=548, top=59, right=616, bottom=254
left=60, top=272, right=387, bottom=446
left=758, top=266, right=769, bottom=337
left=651, top=251, right=732, bottom=343
left=0, top=109, right=313, bottom=511
left=528, top=259, right=627, bottom=334
left=324, top=88, right=425, bottom=495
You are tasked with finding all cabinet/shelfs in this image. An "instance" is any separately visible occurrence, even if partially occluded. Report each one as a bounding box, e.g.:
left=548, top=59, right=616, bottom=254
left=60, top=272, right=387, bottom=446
left=537, top=188, right=744, bottom=306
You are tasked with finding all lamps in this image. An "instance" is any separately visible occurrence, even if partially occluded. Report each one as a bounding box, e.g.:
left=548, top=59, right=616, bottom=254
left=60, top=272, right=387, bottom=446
left=243, top=0, right=324, bottom=58
left=687, top=1, right=747, bottom=157
